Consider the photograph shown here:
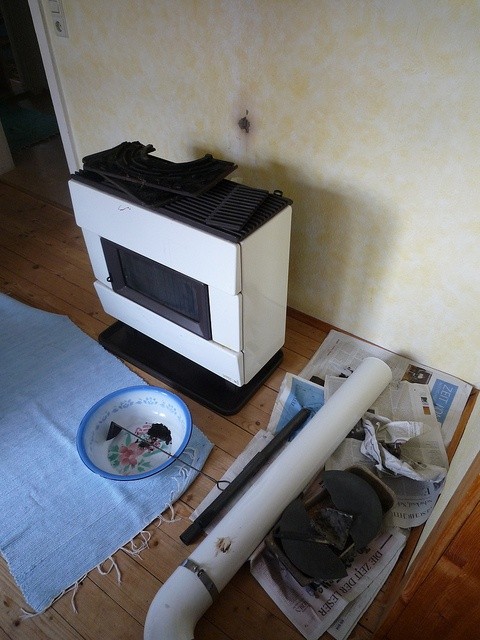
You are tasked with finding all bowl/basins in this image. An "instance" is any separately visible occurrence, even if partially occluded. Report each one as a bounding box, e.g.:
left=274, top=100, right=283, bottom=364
left=76, top=385, right=193, bottom=482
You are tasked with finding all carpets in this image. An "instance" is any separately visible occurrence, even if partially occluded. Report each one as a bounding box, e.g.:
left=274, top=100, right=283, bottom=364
left=0, top=292, right=212, bottom=617
